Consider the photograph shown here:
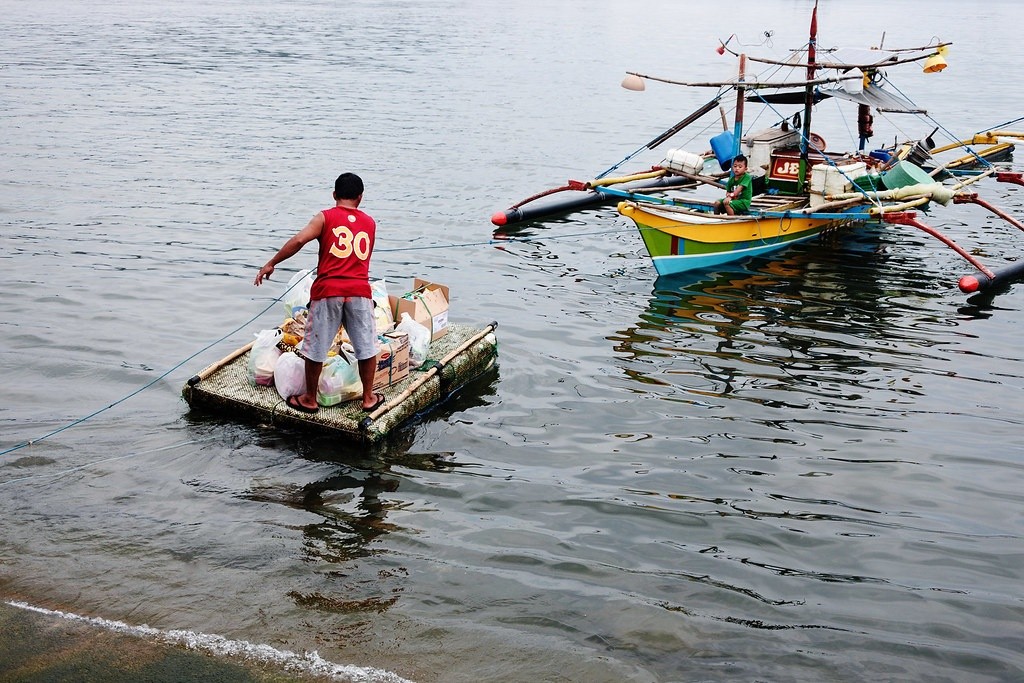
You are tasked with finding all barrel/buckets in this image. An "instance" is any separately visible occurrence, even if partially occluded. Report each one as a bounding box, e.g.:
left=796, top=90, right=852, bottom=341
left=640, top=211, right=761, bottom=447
left=709, top=131, right=745, bottom=171
left=666, top=148, right=704, bottom=176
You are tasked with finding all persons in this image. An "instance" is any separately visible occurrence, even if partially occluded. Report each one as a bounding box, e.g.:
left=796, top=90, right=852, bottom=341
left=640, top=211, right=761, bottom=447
left=713, top=155, right=752, bottom=216
left=254, top=172, right=385, bottom=414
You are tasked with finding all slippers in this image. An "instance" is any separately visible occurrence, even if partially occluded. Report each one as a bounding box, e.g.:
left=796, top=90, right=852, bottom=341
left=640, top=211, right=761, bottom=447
left=363, top=392, right=385, bottom=412
left=286, top=395, right=320, bottom=413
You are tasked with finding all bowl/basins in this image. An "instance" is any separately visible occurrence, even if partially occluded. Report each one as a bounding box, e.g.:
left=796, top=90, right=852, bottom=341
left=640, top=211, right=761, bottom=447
left=881, top=160, right=936, bottom=190
